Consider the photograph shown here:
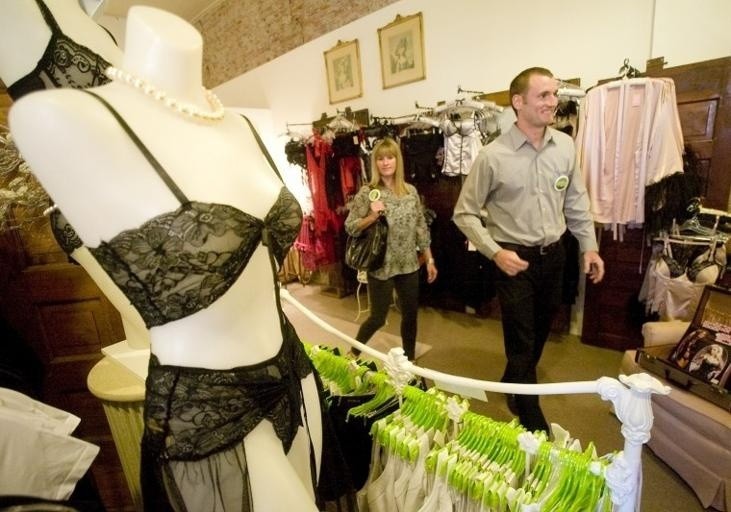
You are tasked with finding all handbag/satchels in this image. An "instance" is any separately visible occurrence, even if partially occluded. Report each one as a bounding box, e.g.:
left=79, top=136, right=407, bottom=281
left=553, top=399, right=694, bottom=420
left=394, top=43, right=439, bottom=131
left=344, top=217, right=388, bottom=270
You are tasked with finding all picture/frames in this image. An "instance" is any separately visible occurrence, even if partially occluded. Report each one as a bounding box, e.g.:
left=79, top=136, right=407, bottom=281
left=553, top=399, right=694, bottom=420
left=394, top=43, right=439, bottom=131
left=376, top=11, right=429, bottom=91
left=323, top=38, right=364, bottom=106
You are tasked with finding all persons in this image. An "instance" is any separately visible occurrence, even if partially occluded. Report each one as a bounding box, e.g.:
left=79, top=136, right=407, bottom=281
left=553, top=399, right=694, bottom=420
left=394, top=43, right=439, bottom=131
left=451, top=68, right=606, bottom=435
left=346, top=138, right=440, bottom=360
left=7, top=6, right=322, bottom=511
left=690, top=345, right=724, bottom=383
left=0, top=0, right=150, bottom=349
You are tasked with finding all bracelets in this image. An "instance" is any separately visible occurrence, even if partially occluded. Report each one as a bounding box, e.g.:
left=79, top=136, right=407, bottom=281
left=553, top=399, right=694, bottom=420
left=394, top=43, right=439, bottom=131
left=426, top=257, right=435, bottom=264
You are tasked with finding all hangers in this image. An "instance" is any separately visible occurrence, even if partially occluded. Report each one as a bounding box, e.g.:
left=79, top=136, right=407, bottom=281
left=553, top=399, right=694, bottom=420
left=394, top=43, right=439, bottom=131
left=602, top=57, right=649, bottom=92
left=646, top=196, right=731, bottom=262
left=300, top=341, right=619, bottom=512
left=283, top=87, right=502, bottom=148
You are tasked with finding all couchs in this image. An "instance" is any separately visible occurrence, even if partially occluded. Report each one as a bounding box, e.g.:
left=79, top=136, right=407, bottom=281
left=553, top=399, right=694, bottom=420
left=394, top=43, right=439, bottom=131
left=610, top=319, right=731, bottom=511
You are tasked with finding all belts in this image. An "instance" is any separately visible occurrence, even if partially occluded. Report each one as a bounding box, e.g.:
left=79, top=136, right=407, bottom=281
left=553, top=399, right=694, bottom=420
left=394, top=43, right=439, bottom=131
left=504, top=243, right=563, bottom=256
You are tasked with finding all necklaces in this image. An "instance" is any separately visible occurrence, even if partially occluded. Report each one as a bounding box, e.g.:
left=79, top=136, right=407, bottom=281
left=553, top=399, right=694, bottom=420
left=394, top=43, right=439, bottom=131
left=102, top=64, right=226, bottom=122
